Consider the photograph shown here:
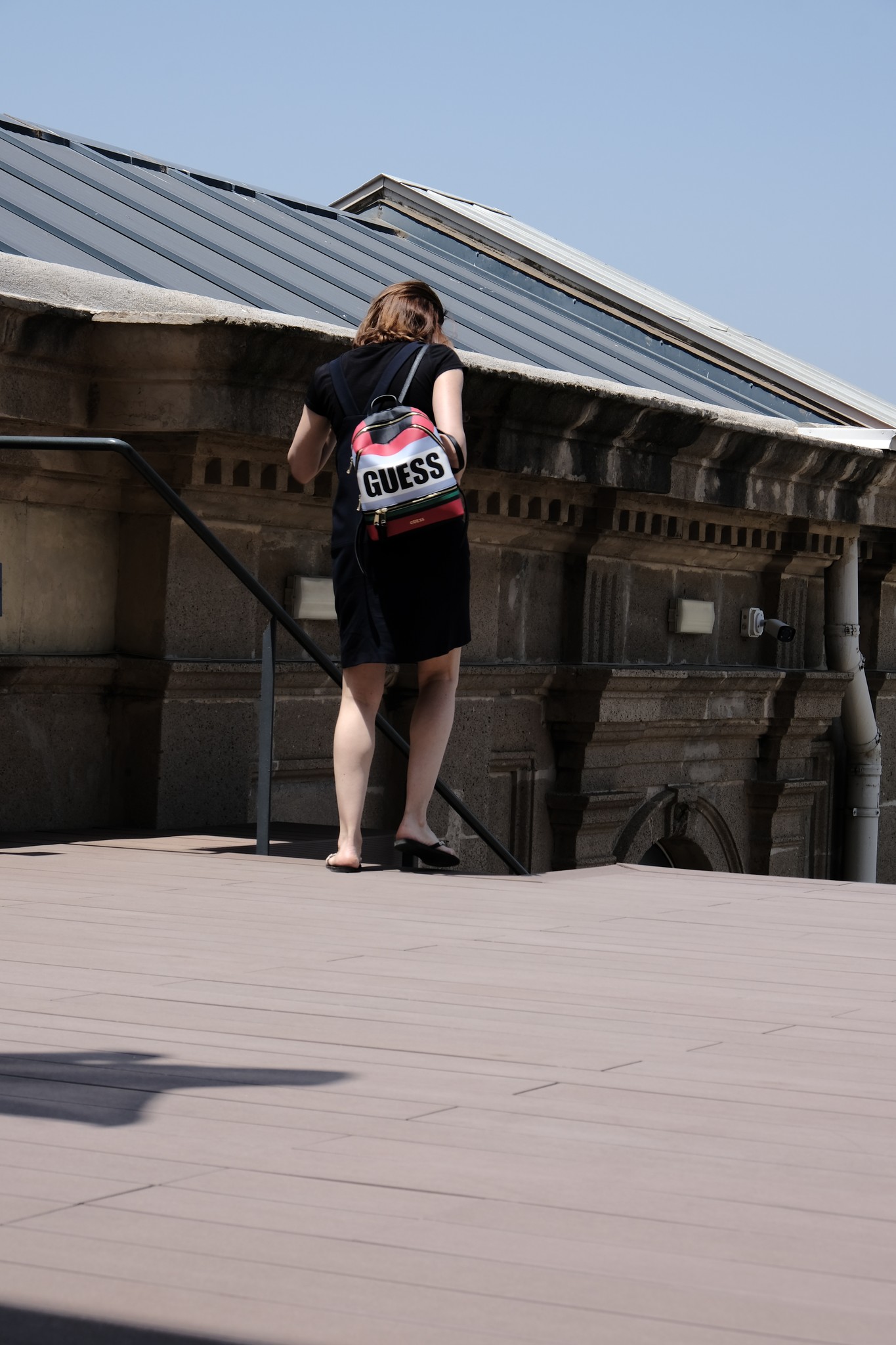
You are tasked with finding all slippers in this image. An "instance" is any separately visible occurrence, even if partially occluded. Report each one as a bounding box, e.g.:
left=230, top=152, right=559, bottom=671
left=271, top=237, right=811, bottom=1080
left=393, top=839, right=460, bottom=867
left=325, top=852, right=362, bottom=873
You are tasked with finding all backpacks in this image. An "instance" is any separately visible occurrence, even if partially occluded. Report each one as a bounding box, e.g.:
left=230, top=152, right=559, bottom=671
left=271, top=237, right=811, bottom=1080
left=346, top=343, right=468, bottom=571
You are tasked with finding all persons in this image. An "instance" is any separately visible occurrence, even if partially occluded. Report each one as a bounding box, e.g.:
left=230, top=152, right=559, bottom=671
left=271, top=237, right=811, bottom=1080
left=288, top=280, right=471, bottom=875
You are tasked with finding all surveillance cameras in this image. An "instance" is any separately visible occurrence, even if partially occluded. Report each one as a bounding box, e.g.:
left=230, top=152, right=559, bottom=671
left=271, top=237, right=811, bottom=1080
left=763, top=619, right=796, bottom=642
